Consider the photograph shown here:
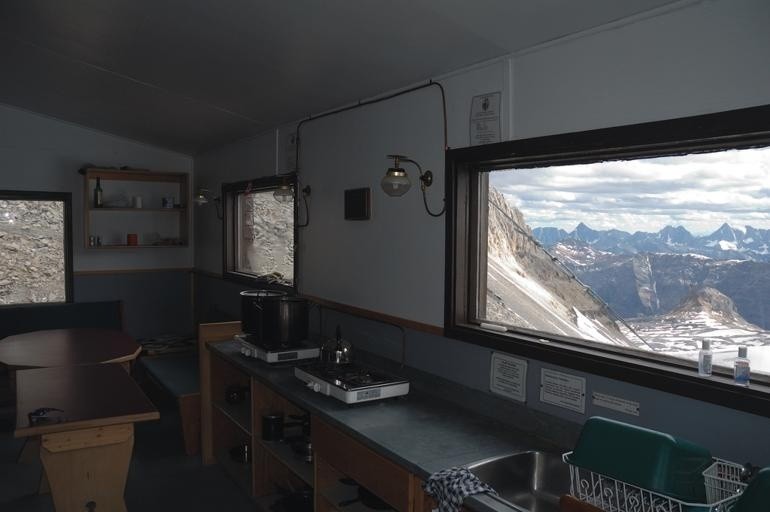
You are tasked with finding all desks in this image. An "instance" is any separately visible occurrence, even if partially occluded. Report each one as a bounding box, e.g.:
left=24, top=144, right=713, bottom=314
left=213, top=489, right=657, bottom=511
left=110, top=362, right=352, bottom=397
left=0, top=328, right=160, bottom=512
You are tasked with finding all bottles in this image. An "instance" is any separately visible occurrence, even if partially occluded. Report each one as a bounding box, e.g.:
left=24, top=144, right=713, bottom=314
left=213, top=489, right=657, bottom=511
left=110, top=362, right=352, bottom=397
left=732, top=347, right=751, bottom=387
left=127, top=232, right=137, bottom=246
left=698, top=340, right=713, bottom=377
left=225, top=382, right=244, bottom=404
left=89, top=235, right=104, bottom=247
left=94, top=177, right=103, bottom=208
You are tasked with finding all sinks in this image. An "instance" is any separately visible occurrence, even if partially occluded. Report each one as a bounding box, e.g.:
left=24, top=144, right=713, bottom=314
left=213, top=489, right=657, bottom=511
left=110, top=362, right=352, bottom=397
left=460, top=449, right=624, bottom=510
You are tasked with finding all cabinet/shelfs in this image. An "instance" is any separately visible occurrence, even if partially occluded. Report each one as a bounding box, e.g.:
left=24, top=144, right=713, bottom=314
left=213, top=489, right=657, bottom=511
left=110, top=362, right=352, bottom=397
left=84, top=167, right=190, bottom=250
left=212, top=356, right=313, bottom=511
left=314, top=419, right=414, bottom=510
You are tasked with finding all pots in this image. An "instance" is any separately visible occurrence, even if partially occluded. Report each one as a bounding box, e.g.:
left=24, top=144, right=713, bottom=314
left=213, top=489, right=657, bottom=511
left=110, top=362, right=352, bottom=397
left=239, top=288, right=310, bottom=342
left=336, top=485, right=393, bottom=511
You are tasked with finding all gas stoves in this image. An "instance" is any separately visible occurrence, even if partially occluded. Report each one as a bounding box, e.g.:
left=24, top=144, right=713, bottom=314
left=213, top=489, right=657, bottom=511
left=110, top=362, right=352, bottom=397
left=234, top=333, right=322, bottom=364
left=294, top=360, right=411, bottom=405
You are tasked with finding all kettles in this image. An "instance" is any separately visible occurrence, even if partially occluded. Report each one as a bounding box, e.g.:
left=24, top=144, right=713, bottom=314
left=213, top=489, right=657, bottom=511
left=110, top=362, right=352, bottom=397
left=319, top=324, right=355, bottom=367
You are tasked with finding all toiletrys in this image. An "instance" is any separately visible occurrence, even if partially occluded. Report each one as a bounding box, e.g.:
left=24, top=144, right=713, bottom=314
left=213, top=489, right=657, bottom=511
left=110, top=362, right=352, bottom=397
left=734, top=347, right=750, bottom=387
left=700, top=341, right=712, bottom=377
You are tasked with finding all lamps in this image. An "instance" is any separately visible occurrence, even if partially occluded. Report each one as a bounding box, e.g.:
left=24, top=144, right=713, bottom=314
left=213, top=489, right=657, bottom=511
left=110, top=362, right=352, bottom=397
left=273, top=185, right=311, bottom=227
left=193, top=184, right=223, bottom=220
left=380, top=155, right=445, bottom=216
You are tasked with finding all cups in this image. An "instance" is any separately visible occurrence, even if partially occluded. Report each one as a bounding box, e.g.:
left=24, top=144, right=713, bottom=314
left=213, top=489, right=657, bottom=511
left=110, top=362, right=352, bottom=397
left=132, top=196, right=142, bottom=208
